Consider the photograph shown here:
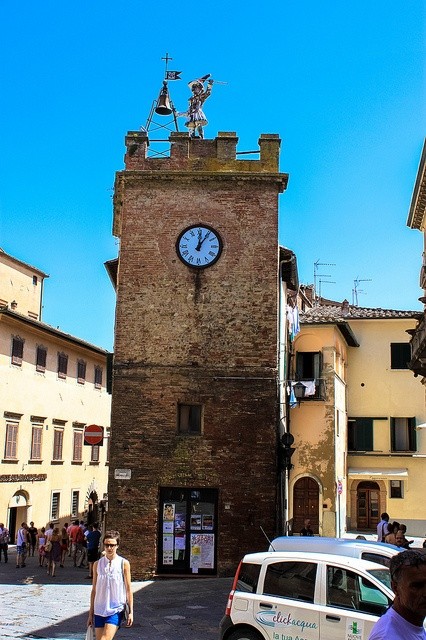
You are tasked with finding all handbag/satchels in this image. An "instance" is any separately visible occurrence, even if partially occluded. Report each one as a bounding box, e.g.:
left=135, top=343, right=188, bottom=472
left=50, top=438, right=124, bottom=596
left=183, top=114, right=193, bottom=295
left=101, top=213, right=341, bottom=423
left=44, top=541, right=53, bottom=552
left=123, top=602, right=130, bottom=623
left=4, top=536, right=10, bottom=543
left=22, top=542, right=26, bottom=548
left=382, top=530, right=387, bottom=543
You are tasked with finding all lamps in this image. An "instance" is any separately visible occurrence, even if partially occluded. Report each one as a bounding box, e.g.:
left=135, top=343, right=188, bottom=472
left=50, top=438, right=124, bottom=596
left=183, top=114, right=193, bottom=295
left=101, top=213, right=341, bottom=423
left=282, top=379, right=306, bottom=408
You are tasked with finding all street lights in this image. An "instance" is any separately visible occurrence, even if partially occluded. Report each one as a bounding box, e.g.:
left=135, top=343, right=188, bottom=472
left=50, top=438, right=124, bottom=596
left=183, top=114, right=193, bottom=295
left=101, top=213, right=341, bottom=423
left=282, top=381, right=305, bottom=469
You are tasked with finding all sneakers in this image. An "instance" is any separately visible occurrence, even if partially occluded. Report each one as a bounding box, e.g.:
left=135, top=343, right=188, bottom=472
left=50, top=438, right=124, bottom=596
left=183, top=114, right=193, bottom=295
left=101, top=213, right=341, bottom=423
left=86, top=576, right=92, bottom=579
left=21, top=564, right=25, bottom=567
left=16, top=565, right=19, bottom=568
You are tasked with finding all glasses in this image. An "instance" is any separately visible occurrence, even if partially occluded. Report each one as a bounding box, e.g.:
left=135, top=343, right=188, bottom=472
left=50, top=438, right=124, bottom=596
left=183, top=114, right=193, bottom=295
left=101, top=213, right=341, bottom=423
left=104, top=543, right=118, bottom=547
left=391, top=554, right=426, bottom=580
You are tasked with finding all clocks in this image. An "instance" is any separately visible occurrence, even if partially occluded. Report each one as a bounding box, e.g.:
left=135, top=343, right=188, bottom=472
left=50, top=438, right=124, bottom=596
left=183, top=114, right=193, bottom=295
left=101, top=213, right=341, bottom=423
left=175, top=224, right=223, bottom=268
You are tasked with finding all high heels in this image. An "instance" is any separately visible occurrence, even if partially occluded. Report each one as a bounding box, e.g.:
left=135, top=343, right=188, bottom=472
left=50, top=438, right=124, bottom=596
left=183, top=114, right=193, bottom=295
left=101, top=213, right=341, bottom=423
left=59, top=564, right=61, bottom=567
left=62, top=564, right=63, bottom=568
left=40, top=565, right=43, bottom=567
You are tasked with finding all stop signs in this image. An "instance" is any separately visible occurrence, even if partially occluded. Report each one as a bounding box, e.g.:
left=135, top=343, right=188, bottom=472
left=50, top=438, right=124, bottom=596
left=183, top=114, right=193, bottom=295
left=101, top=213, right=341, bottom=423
left=84, top=425, right=102, bottom=445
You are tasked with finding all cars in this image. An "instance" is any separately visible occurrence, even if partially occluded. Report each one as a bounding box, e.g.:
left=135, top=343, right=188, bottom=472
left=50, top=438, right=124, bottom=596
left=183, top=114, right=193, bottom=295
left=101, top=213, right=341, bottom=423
left=220, top=552, right=426, bottom=640
left=268, top=536, right=407, bottom=567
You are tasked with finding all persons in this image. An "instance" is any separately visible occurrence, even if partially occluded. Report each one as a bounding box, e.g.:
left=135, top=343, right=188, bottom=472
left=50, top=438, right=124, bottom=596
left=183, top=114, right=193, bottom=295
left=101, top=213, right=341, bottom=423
left=367, top=550, right=426, bottom=640
left=28, top=522, right=38, bottom=557
left=79, top=520, right=85, bottom=532
left=84, top=522, right=101, bottom=579
left=385, top=521, right=414, bottom=550
left=164, top=504, right=173, bottom=520
left=0, top=523, right=10, bottom=564
left=376, top=513, right=389, bottom=542
left=300, top=519, right=314, bottom=536
left=70, top=520, right=87, bottom=568
left=67, top=521, right=76, bottom=557
left=15, top=522, right=27, bottom=568
left=87, top=530, right=133, bottom=640
left=64, top=522, right=72, bottom=557
left=83, top=525, right=93, bottom=568
left=37, top=527, right=48, bottom=567
left=59, top=528, right=69, bottom=568
left=47, top=528, right=61, bottom=577
left=45, top=522, right=55, bottom=566
